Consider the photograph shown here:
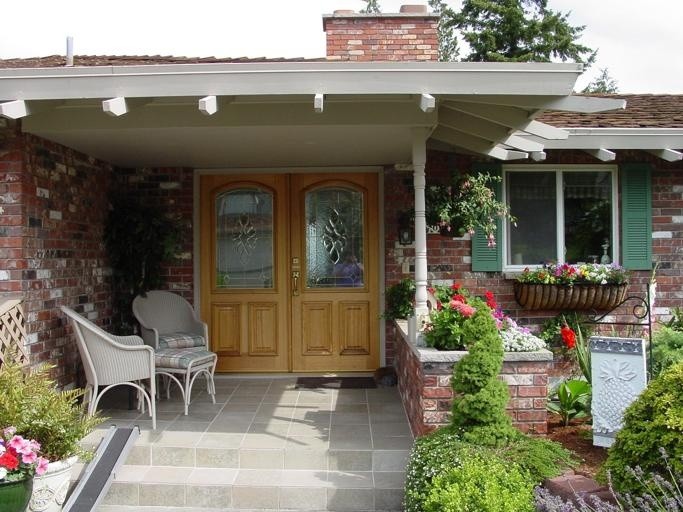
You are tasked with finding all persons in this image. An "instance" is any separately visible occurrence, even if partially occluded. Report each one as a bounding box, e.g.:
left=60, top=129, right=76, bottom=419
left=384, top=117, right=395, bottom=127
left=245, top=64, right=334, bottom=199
left=333, top=250, right=364, bottom=287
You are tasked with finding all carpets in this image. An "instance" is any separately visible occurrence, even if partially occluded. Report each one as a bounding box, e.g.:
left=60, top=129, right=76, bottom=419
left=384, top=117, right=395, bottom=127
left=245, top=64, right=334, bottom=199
left=294, top=377, right=378, bottom=389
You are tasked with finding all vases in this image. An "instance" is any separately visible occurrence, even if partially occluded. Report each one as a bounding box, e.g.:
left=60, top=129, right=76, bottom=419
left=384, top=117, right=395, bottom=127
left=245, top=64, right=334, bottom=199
left=0, top=477, right=33, bottom=512
left=439, top=214, right=463, bottom=237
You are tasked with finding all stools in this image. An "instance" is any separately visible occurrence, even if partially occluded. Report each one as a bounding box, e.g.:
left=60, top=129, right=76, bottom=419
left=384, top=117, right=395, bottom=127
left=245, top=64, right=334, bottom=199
left=141, top=351, right=217, bottom=416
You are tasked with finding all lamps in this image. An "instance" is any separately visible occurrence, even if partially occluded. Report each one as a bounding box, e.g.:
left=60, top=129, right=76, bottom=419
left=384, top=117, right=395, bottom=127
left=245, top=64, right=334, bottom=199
left=399, top=226, right=412, bottom=245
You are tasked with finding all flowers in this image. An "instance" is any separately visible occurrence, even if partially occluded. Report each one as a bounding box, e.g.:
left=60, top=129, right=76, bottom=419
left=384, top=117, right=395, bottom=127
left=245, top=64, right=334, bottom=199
left=514, top=261, right=632, bottom=287
left=0, top=427, right=49, bottom=480
left=421, top=172, right=519, bottom=249
left=379, top=281, right=546, bottom=351
left=540, top=310, right=593, bottom=351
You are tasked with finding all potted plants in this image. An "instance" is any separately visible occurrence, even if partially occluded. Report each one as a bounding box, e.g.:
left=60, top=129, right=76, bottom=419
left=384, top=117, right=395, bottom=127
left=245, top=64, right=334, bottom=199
left=0, top=346, right=111, bottom=512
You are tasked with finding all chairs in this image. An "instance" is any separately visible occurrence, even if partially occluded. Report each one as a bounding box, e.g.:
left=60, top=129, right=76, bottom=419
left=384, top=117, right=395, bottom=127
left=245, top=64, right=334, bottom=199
left=132, top=290, right=209, bottom=400
left=60, top=305, right=156, bottom=431
